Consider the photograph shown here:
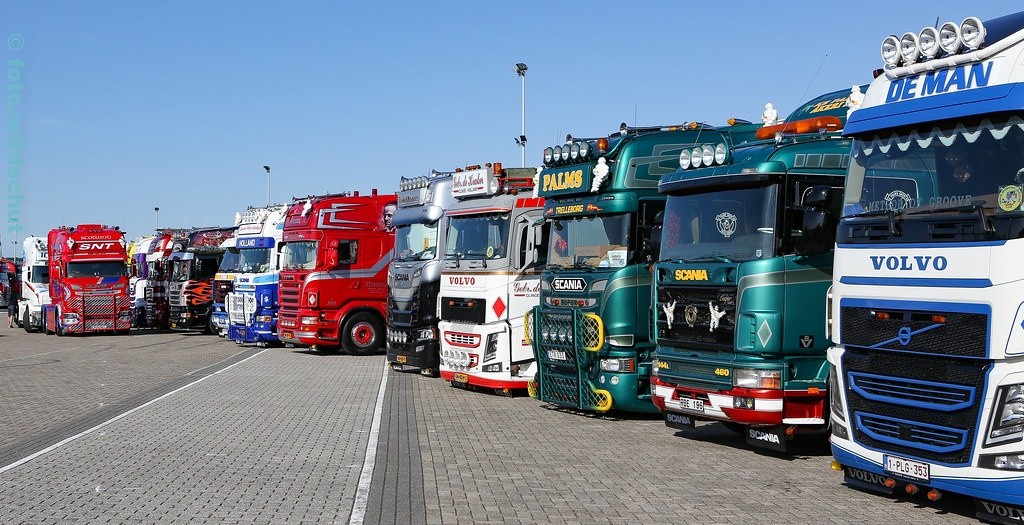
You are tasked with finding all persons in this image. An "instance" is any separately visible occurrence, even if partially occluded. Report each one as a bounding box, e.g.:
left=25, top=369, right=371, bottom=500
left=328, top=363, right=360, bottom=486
left=7, top=299, right=20, bottom=328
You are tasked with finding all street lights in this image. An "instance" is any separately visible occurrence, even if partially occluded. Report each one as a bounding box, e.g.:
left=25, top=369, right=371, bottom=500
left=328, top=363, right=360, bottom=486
left=513, top=62, right=528, bottom=168
left=11, top=218, right=19, bottom=266
left=154, top=206, right=160, bottom=235
left=0, top=235, right=4, bottom=258
left=263, top=164, right=271, bottom=206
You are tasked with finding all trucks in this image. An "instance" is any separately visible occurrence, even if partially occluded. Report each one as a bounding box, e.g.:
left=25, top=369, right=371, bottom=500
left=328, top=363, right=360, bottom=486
left=816, top=12, right=1024, bottom=525
left=211, top=201, right=315, bottom=348
left=384, top=163, right=483, bottom=378
left=42, top=222, right=133, bottom=337
left=649, top=83, right=956, bottom=452
left=0, top=258, right=21, bottom=309
left=14, top=235, right=52, bottom=333
left=435, top=161, right=578, bottom=397
left=126, top=224, right=237, bottom=333
left=276, top=188, right=437, bottom=356
left=522, top=115, right=794, bottom=414
